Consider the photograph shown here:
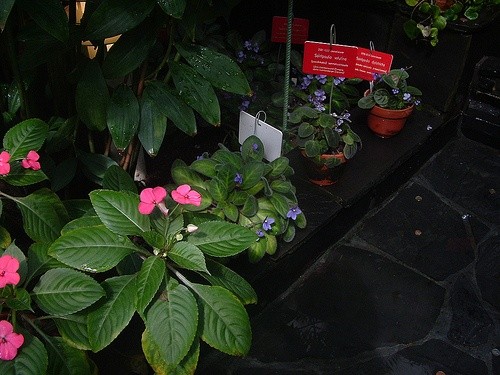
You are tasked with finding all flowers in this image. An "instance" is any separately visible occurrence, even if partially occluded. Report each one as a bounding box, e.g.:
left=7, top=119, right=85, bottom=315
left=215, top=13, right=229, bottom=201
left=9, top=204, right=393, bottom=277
left=0, top=0, right=479, bottom=375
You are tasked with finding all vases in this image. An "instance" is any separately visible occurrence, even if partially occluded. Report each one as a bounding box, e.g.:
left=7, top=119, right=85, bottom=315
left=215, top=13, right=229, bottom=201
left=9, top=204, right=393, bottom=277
left=365, top=89, right=416, bottom=138
left=301, top=150, right=352, bottom=166
